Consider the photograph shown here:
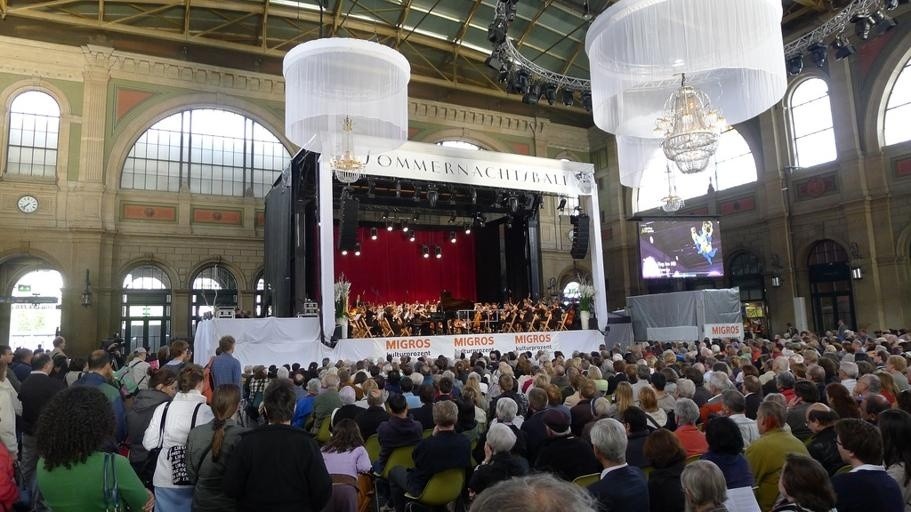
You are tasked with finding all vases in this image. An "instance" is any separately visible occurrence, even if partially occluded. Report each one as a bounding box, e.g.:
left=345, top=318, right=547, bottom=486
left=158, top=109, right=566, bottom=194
left=337, top=314, right=349, bottom=339
left=581, top=309, right=591, bottom=330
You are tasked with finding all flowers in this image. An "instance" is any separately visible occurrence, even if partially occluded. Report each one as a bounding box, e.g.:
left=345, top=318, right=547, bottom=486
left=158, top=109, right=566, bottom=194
left=579, top=278, right=598, bottom=312
left=333, top=278, right=352, bottom=318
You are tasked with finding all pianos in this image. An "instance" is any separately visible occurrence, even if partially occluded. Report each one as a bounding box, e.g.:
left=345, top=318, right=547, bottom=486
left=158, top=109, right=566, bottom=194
left=426, top=291, right=473, bottom=335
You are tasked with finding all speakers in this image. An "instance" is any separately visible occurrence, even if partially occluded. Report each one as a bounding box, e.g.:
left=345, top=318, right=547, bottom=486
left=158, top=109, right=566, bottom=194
left=337, top=199, right=359, bottom=251
left=589, top=318, right=598, bottom=329
left=571, top=215, right=589, bottom=259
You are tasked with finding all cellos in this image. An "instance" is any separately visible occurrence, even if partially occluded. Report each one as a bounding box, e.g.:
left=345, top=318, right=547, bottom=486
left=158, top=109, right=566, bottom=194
left=565, top=297, right=575, bottom=324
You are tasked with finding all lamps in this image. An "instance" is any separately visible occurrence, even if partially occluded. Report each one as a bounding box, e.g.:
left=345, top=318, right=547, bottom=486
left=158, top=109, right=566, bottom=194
left=283, top=0, right=411, bottom=185
left=615, top=114, right=732, bottom=212
left=768, top=255, right=782, bottom=290
left=341, top=176, right=568, bottom=258
left=849, top=243, right=867, bottom=281
left=585, top=1, right=787, bottom=173
left=486, top=1, right=911, bottom=115
left=81, top=269, right=94, bottom=309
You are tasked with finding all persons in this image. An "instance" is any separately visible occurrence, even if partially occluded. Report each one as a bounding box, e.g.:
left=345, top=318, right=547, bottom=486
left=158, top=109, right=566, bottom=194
left=345, top=300, right=447, bottom=338
left=0, top=337, right=910, bottom=512
left=448, top=298, right=568, bottom=334
left=690, top=221, right=718, bottom=266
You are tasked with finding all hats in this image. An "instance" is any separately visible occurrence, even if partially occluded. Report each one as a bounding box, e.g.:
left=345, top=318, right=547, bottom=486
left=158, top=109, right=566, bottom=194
left=541, top=407, right=572, bottom=426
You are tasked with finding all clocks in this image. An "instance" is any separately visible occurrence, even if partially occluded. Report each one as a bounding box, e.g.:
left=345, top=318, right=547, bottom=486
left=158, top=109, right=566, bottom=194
left=16, top=195, right=39, bottom=213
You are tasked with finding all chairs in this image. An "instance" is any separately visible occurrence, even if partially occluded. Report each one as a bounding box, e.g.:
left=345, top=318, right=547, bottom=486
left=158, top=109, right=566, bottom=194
left=315, top=368, right=895, bottom=512
left=354, top=304, right=572, bottom=336
